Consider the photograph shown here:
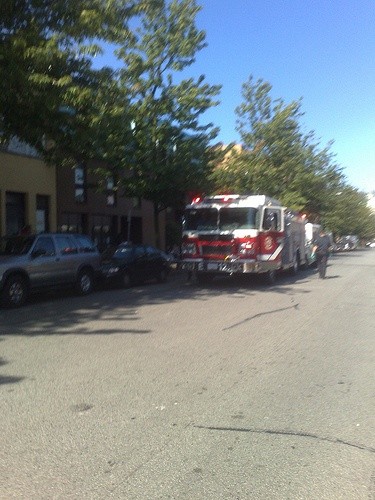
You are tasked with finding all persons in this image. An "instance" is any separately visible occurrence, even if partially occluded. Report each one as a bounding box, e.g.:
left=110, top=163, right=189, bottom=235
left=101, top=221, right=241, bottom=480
left=311, top=231, right=331, bottom=280
left=164, top=244, right=181, bottom=262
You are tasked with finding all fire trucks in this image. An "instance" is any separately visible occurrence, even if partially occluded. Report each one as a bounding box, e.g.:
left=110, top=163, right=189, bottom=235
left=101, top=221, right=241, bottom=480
left=178, top=194, right=304, bottom=284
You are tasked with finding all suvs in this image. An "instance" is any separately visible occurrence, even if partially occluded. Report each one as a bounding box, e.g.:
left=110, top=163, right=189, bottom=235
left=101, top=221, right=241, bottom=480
left=0, top=232, right=102, bottom=309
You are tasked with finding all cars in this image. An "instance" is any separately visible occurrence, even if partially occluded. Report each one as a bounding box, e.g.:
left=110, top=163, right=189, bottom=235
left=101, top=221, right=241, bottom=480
left=100, top=245, right=171, bottom=289
